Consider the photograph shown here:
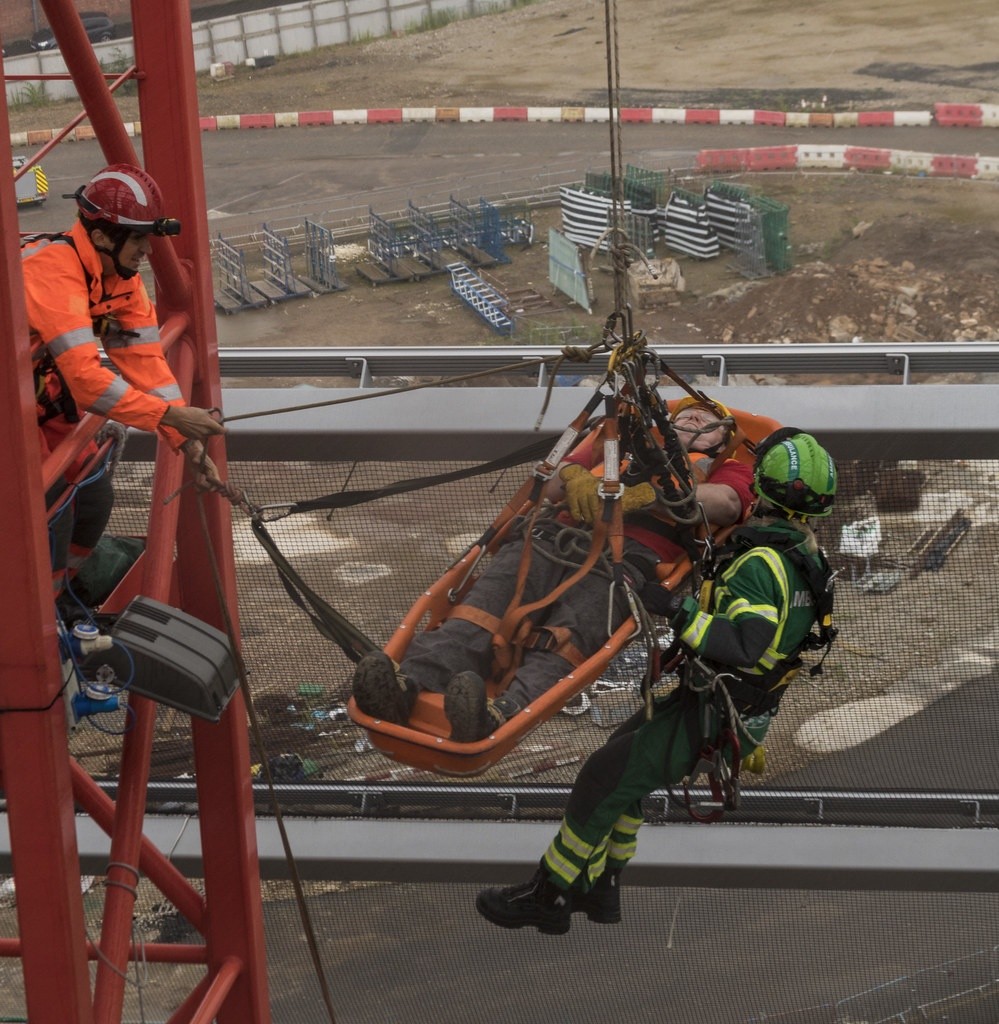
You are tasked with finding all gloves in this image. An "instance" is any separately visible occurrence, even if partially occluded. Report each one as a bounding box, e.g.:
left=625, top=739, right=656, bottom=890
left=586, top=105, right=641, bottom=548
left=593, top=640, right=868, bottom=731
left=559, top=462, right=605, bottom=524
left=641, top=581, right=683, bottom=621
left=600, top=481, right=655, bottom=512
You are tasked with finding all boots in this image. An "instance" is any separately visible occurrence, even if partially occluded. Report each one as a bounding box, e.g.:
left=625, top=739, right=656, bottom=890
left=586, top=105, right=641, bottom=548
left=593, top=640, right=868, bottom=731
left=571, top=865, right=622, bottom=923
left=443, top=671, right=521, bottom=743
left=352, top=650, right=417, bottom=724
left=475, top=857, right=570, bottom=934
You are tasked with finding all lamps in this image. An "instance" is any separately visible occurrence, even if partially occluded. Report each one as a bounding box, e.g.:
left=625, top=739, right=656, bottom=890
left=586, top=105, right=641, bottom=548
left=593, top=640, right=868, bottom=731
left=93, top=594, right=247, bottom=718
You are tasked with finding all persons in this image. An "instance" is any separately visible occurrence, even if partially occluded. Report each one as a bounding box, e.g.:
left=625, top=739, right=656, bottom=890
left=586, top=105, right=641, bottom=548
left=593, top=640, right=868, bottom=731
left=352, top=392, right=754, bottom=743
left=18, top=162, right=227, bottom=600
left=472, top=428, right=842, bottom=934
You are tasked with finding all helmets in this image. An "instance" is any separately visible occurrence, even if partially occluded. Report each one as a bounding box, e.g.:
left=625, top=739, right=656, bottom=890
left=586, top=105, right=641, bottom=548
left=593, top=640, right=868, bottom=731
left=669, top=396, right=734, bottom=442
left=754, top=426, right=837, bottom=516
left=75, top=163, right=166, bottom=235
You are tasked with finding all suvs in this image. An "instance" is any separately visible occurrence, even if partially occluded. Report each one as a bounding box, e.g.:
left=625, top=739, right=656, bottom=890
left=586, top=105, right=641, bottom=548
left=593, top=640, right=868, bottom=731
left=28, top=10, right=115, bottom=52
